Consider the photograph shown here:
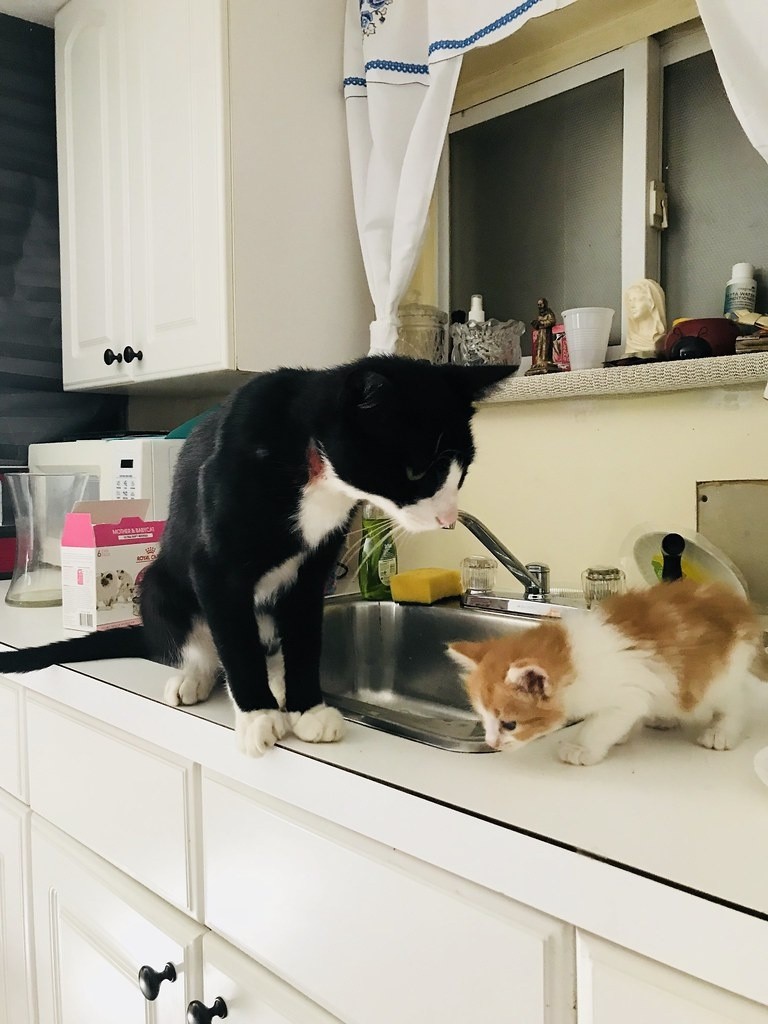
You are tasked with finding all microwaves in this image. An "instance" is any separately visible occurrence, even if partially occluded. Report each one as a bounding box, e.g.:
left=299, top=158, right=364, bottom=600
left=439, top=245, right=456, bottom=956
left=27, top=438, right=186, bottom=569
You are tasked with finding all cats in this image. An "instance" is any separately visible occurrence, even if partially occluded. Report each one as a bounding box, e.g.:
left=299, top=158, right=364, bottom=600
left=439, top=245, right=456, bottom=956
left=441, top=576, right=765, bottom=766
left=0, top=352, right=520, bottom=761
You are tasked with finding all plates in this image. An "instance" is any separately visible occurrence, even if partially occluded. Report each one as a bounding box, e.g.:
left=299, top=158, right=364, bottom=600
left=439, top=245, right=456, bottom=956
left=630, top=531, right=750, bottom=611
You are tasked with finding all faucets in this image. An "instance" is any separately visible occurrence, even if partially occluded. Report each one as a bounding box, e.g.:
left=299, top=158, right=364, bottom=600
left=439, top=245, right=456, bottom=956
left=453, top=507, right=546, bottom=602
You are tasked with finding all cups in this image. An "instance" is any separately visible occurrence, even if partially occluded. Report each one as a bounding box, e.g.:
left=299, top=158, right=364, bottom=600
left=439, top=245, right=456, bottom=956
left=398, top=289, right=449, bottom=364
left=561, top=307, right=614, bottom=369
left=4, top=474, right=88, bottom=607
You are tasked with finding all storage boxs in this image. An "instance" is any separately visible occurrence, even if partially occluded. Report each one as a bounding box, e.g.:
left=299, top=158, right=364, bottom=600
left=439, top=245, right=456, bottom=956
left=60, top=498, right=167, bottom=634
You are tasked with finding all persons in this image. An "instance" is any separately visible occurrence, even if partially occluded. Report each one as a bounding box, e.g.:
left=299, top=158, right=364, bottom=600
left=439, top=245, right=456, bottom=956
left=622, top=277, right=667, bottom=358
left=531, top=297, right=556, bottom=367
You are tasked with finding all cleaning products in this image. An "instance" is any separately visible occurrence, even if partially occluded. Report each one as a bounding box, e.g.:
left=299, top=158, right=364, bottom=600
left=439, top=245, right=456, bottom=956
left=467, top=293, right=484, bottom=323
left=722, top=262, right=757, bottom=322
left=356, top=503, right=399, bottom=597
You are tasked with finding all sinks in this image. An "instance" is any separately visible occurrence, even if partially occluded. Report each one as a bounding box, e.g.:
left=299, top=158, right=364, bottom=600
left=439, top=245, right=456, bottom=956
left=313, top=599, right=533, bottom=716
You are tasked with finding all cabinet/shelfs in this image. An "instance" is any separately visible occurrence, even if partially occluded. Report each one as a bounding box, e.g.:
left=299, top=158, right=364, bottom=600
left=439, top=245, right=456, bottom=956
left=54, top=1, right=375, bottom=397
left=1, top=677, right=768, bottom=1024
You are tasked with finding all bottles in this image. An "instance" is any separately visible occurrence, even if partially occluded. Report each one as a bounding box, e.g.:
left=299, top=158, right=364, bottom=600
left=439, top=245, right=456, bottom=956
left=723, top=263, right=758, bottom=319
